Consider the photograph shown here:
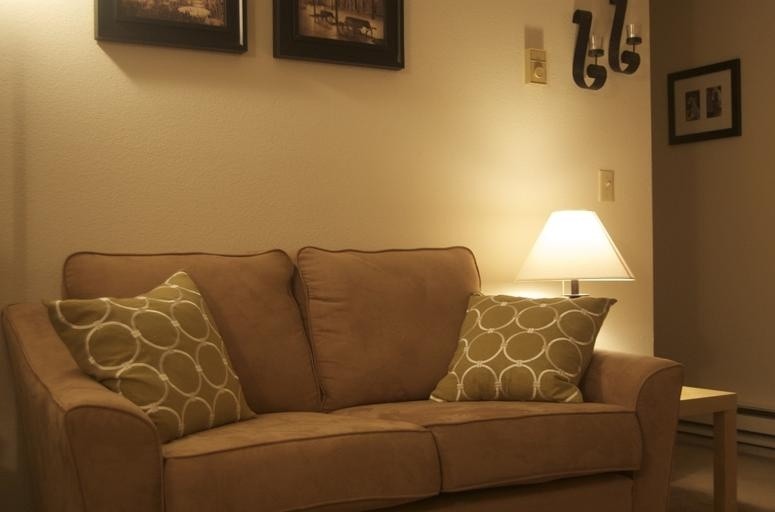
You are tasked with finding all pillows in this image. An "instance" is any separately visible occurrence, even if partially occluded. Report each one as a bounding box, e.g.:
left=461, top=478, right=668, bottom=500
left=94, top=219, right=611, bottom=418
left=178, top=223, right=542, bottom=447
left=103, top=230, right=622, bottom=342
left=427, top=292, right=619, bottom=403
left=41, top=269, right=258, bottom=446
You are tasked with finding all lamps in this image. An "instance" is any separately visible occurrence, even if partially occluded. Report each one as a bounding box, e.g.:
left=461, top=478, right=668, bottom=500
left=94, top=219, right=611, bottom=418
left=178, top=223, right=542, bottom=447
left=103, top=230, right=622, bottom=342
left=572, top=0, right=643, bottom=89
left=516, top=210, right=636, bottom=298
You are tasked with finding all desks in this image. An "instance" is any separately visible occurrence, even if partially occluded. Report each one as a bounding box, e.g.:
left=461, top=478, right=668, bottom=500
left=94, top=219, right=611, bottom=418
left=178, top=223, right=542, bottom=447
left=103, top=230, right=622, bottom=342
left=678, top=385, right=738, bottom=511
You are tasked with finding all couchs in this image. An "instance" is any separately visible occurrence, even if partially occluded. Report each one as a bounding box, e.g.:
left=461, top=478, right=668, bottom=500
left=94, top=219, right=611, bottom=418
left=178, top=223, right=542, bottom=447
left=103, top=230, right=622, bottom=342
left=0, top=246, right=687, bottom=511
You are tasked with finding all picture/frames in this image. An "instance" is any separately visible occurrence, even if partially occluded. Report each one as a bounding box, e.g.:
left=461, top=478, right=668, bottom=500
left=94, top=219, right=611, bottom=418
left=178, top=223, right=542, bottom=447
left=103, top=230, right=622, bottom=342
left=667, top=58, right=743, bottom=146
left=273, top=2, right=404, bottom=72
left=93, top=2, right=248, bottom=55
